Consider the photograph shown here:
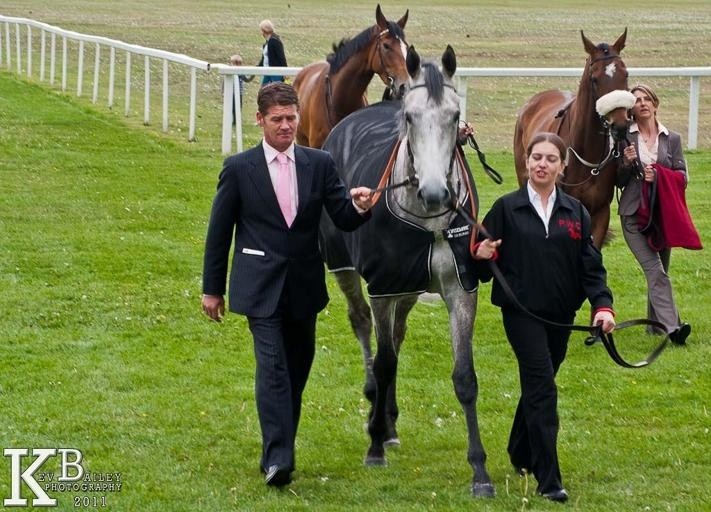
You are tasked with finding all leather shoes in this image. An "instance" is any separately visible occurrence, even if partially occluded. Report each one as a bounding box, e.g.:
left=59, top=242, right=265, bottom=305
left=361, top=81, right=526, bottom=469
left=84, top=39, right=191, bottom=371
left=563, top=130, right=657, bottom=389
left=263, top=465, right=291, bottom=487
left=669, top=322, right=691, bottom=344
left=535, top=487, right=568, bottom=501
left=511, top=453, right=531, bottom=475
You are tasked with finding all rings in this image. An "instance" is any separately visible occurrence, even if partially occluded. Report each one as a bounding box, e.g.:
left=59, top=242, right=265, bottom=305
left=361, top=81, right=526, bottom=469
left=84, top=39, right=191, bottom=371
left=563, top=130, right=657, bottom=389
left=627, top=150, right=631, bottom=153
left=487, top=252, right=491, bottom=258
left=212, top=312, right=218, bottom=316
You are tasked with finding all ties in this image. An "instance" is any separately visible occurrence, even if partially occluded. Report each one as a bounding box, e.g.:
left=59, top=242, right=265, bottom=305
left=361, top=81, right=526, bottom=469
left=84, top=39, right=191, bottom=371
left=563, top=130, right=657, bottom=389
left=276, top=153, right=292, bottom=229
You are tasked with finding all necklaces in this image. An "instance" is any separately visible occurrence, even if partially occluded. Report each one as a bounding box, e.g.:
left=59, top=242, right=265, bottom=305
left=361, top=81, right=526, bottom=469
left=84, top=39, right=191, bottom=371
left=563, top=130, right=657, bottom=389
left=639, top=127, right=656, bottom=143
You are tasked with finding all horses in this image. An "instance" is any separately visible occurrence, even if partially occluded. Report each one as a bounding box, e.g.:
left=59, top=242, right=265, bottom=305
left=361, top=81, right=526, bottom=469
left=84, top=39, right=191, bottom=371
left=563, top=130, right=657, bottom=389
left=318, top=44, right=497, bottom=499
left=291, top=3, right=410, bottom=149
left=513, top=26, right=630, bottom=253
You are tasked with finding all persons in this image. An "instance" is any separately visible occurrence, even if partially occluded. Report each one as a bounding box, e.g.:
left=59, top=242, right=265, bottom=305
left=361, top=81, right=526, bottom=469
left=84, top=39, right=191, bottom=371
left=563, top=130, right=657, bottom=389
left=615, top=84, right=690, bottom=345
left=200, top=81, right=373, bottom=487
left=256, top=18, right=290, bottom=86
left=218, top=54, right=250, bottom=124
left=474, top=132, right=615, bottom=501
